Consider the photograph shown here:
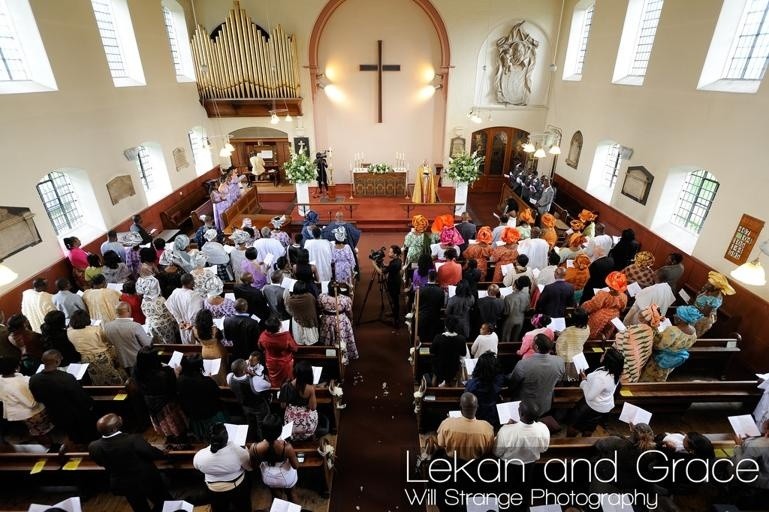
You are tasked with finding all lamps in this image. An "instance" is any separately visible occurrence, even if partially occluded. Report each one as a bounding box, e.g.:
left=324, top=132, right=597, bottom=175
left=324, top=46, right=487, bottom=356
left=467, top=17, right=496, bottom=123
left=316, top=72, right=324, bottom=80
left=521, top=1, right=572, bottom=159
left=433, top=84, right=443, bottom=90
left=317, top=81, right=325, bottom=89
left=433, top=73, right=443, bottom=79
left=261, top=5, right=292, bottom=124
left=190, top=0, right=235, bottom=157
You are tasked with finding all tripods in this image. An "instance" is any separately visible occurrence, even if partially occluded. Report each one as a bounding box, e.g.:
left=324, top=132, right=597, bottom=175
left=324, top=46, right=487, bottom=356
left=359, top=263, right=396, bottom=332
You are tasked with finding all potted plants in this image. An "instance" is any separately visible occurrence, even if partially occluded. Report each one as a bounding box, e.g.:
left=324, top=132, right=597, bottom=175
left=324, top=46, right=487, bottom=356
left=443, top=149, right=487, bottom=214
left=282, top=144, right=317, bottom=215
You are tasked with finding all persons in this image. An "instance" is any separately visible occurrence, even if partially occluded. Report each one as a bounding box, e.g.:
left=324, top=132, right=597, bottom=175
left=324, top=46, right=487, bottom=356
left=311, top=151, right=330, bottom=193
left=249, top=149, right=267, bottom=181
left=370, top=245, right=402, bottom=330
left=401, top=157, right=768, bottom=512
left=0, top=168, right=362, bottom=512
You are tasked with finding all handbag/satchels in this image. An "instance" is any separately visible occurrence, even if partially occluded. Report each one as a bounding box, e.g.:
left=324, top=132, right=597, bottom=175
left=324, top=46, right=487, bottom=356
left=280, top=457, right=290, bottom=471
left=254, top=413, right=282, bottom=443
left=284, top=405, right=309, bottom=434
